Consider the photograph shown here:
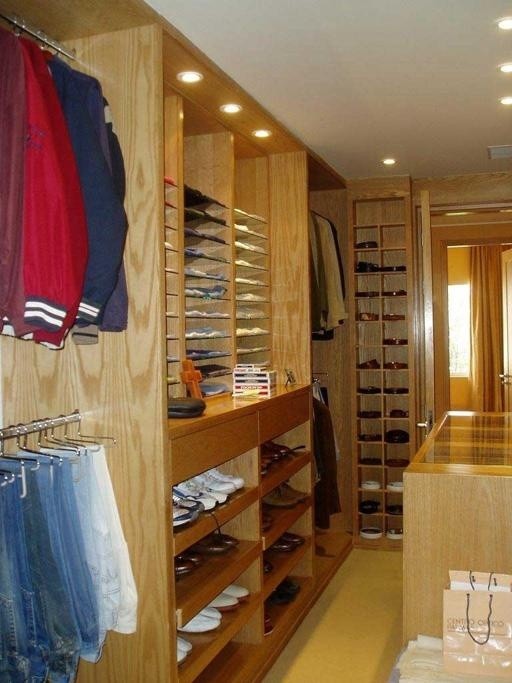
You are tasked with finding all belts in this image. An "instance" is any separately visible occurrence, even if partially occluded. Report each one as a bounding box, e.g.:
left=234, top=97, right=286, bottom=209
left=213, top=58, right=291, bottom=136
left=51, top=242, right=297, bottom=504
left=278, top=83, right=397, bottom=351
left=355, top=260, right=407, bottom=321
left=386, top=503, right=403, bottom=516
left=387, top=480, right=403, bottom=494
left=386, top=527, right=404, bottom=540
left=386, top=457, right=409, bottom=468
left=385, top=429, right=409, bottom=445
left=359, top=526, right=383, bottom=540
left=355, top=240, right=377, bottom=248
left=359, top=479, right=382, bottom=491
left=383, top=336, right=408, bottom=345
left=359, top=499, right=381, bottom=515
left=359, top=433, right=382, bottom=442
left=357, top=357, right=408, bottom=418
left=359, top=457, right=381, bottom=466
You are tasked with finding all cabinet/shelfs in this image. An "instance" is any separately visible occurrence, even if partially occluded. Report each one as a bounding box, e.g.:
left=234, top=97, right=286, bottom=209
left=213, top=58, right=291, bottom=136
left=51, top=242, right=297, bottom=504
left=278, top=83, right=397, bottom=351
left=348, top=175, right=417, bottom=551
left=0, top=0, right=355, bottom=683
left=404, top=410, right=512, bottom=649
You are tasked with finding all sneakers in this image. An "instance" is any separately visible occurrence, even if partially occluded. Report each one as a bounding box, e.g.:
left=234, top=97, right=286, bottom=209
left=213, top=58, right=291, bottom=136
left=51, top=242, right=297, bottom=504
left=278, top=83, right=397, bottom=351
left=206, top=468, right=246, bottom=491
left=182, top=479, right=230, bottom=505
left=170, top=500, right=199, bottom=529
left=193, top=473, right=237, bottom=495
left=172, top=488, right=204, bottom=514
left=176, top=483, right=219, bottom=512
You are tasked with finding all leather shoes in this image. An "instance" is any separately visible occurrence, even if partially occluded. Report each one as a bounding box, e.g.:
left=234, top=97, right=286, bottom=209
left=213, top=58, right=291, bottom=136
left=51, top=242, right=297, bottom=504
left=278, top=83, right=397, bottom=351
left=191, top=538, right=233, bottom=557
left=176, top=636, right=193, bottom=656
left=261, top=490, right=299, bottom=509
left=261, top=513, right=274, bottom=534
left=175, top=614, right=222, bottom=634
left=264, top=576, right=302, bottom=635
left=176, top=649, right=188, bottom=667
left=177, top=550, right=202, bottom=563
left=200, top=607, right=223, bottom=621
left=204, top=593, right=241, bottom=612
left=222, top=583, right=251, bottom=603
left=173, top=557, right=195, bottom=578
left=203, top=534, right=241, bottom=547
left=263, top=532, right=305, bottom=573
left=259, top=441, right=293, bottom=476
left=274, top=481, right=310, bottom=503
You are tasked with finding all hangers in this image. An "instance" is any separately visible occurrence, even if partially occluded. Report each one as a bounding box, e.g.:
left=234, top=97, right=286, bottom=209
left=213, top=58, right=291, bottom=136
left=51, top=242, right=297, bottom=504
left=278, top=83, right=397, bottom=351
left=10, top=12, right=101, bottom=88
left=0, top=411, right=118, bottom=485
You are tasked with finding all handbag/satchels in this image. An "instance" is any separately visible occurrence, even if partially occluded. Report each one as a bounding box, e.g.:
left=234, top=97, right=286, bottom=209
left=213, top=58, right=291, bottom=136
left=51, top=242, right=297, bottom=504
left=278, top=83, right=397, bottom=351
left=443, top=567, right=512, bottom=681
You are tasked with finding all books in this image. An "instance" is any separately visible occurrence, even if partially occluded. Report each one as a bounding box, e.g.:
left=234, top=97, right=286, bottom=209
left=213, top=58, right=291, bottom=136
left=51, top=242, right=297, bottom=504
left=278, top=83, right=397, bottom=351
left=231, top=362, right=278, bottom=396
